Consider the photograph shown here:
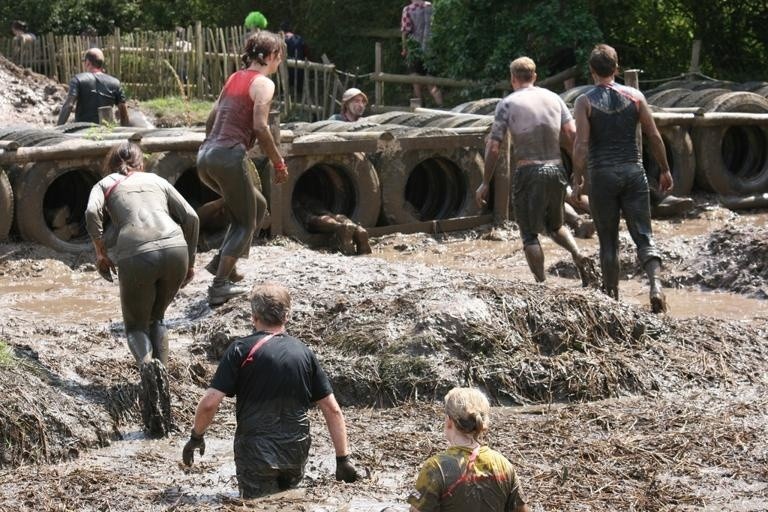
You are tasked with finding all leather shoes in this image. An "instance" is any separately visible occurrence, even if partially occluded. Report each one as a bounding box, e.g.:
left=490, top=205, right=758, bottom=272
left=205, top=250, right=243, bottom=282
left=208, top=281, right=249, bottom=304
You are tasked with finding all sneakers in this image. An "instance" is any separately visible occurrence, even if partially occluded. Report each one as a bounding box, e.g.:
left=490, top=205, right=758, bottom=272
left=336, top=223, right=356, bottom=255
left=577, top=257, right=592, bottom=287
left=650, top=287, right=666, bottom=312
left=356, top=226, right=372, bottom=255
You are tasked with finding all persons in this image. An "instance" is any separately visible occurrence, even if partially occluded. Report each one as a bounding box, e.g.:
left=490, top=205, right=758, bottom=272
left=475, top=55, right=599, bottom=288
left=278, top=22, right=308, bottom=100
left=401, top=1, right=446, bottom=109
left=85, top=142, right=200, bottom=440
left=10, top=20, right=42, bottom=74
left=405, top=387, right=532, bottom=511
left=57, top=47, right=129, bottom=128
left=195, top=30, right=289, bottom=303
left=45, top=205, right=80, bottom=241
left=572, top=44, right=675, bottom=315
left=329, top=87, right=368, bottom=123
left=181, top=283, right=363, bottom=502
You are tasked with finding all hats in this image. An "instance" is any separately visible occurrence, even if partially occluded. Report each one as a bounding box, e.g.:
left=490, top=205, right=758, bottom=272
left=342, top=88, right=369, bottom=105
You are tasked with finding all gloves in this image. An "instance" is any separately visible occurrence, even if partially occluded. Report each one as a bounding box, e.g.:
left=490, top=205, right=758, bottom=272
left=183, top=429, right=205, bottom=467
left=273, top=161, right=288, bottom=184
left=336, top=454, right=357, bottom=483
